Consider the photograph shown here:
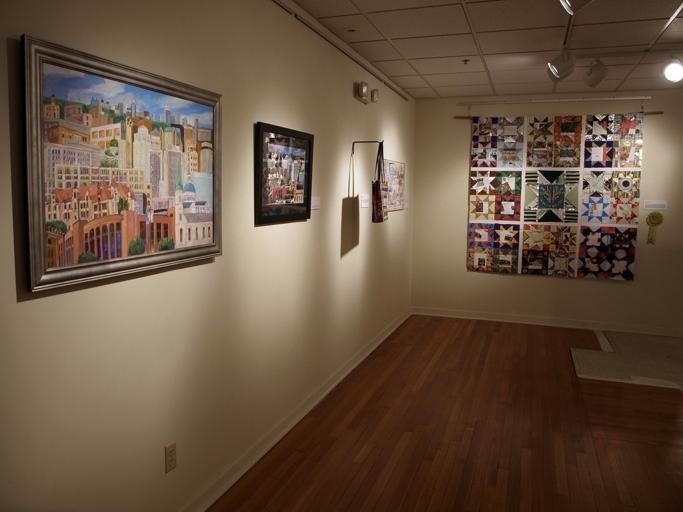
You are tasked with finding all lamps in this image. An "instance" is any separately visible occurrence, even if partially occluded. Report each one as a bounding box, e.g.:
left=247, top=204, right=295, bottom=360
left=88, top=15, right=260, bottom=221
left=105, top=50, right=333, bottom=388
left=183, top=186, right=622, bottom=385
left=352, top=78, right=380, bottom=106
left=572, top=47, right=683, bottom=90
left=544, top=15, right=578, bottom=81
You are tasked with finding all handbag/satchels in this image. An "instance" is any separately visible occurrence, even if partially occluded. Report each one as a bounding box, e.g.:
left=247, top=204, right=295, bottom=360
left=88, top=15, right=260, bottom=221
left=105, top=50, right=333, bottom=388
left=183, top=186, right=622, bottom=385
left=369, top=140, right=390, bottom=224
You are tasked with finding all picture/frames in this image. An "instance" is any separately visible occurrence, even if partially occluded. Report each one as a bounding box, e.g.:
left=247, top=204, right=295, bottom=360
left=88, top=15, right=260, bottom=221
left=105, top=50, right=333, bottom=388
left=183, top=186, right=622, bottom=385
left=19, top=29, right=222, bottom=297
left=253, top=118, right=312, bottom=225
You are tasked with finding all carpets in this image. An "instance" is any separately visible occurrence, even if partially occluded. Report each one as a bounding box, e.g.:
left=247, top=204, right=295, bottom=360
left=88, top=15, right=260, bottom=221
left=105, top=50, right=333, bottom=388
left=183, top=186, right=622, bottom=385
left=564, top=329, right=682, bottom=392
